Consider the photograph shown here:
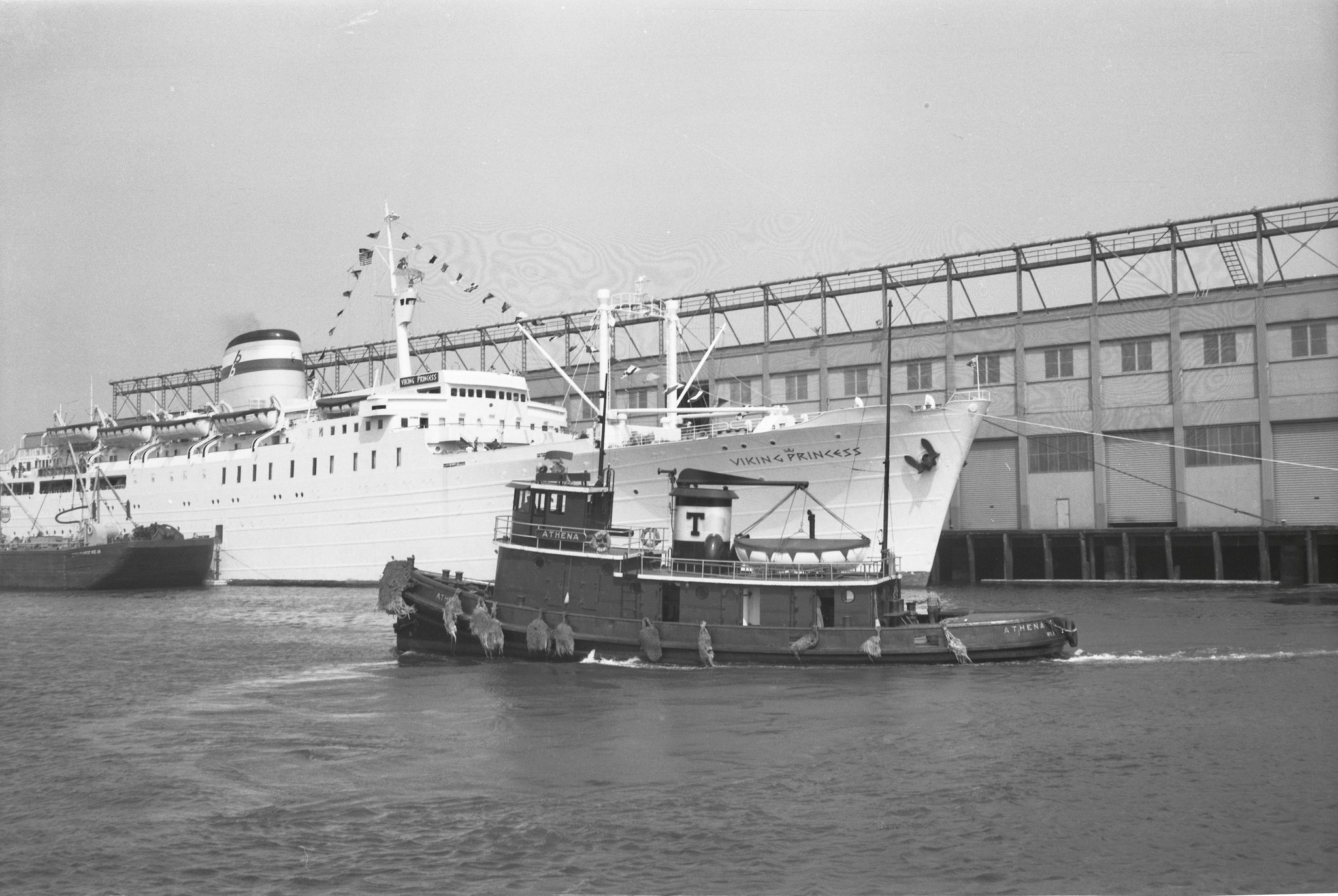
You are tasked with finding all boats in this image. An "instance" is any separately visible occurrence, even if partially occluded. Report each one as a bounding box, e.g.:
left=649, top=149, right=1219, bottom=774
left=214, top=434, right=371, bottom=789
left=0, top=441, right=222, bottom=593
left=0, top=192, right=995, bottom=589
left=96, top=418, right=152, bottom=447
left=212, top=406, right=281, bottom=435
left=731, top=534, right=871, bottom=553
left=152, top=409, right=212, bottom=441
left=389, top=297, right=1078, bottom=670
left=48, top=421, right=102, bottom=446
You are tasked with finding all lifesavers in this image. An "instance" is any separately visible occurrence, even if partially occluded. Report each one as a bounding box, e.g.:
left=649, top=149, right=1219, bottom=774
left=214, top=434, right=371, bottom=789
left=641, top=527, right=660, bottom=548
left=593, top=531, right=611, bottom=552
left=87, top=525, right=93, bottom=535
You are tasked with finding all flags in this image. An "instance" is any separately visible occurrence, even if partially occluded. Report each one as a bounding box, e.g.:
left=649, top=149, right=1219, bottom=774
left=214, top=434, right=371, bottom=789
left=307, top=231, right=751, bottom=421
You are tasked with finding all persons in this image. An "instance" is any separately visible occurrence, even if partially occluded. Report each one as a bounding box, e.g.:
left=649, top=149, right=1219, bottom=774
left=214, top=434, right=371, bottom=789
left=521, top=496, right=537, bottom=515
left=916, top=587, right=943, bottom=623
left=11, top=464, right=27, bottom=478
